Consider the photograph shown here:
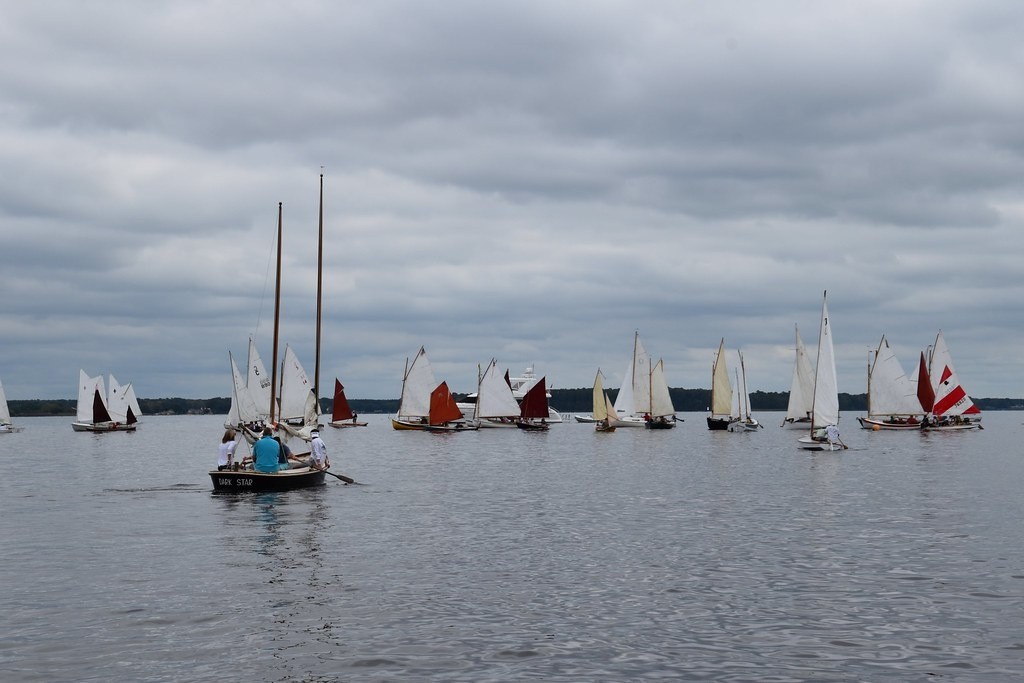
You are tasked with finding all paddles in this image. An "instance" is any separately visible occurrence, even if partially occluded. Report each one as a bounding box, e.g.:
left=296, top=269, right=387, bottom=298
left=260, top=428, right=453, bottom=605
left=676, top=418, right=686, bottom=422
left=837, top=437, right=849, bottom=449
left=758, top=423, right=764, bottom=429
left=290, top=456, right=354, bottom=484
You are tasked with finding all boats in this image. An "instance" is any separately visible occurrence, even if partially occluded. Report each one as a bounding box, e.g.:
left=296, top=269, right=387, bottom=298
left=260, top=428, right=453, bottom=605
left=456, top=357, right=562, bottom=430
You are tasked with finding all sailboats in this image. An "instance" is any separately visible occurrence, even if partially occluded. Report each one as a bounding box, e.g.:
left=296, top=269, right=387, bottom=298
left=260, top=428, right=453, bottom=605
left=0, top=380, right=24, bottom=433
left=856, top=334, right=926, bottom=430
left=391, top=345, right=438, bottom=430
left=781, top=321, right=815, bottom=430
left=908, top=329, right=982, bottom=431
left=72, top=368, right=143, bottom=431
left=706, top=337, right=759, bottom=432
left=424, top=381, right=478, bottom=431
left=327, top=378, right=368, bottom=429
left=207, top=175, right=332, bottom=494
left=797, top=289, right=843, bottom=451
left=574, top=328, right=677, bottom=433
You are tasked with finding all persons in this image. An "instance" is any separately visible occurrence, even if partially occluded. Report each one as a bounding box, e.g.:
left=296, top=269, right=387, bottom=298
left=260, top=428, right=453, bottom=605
left=642, top=413, right=667, bottom=423
left=826, top=425, right=839, bottom=443
left=309, top=429, right=327, bottom=470
left=729, top=415, right=752, bottom=423
left=217, top=428, right=238, bottom=471
left=252, top=427, right=295, bottom=474
left=421, top=417, right=427, bottom=424
left=352, top=411, right=357, bottom=424
left=499, top=416, right=507, bottom=423
left=886, top=414, right=971, bottom=426
left=250, top=421, right=264, bottom=429
left=524, top=418, right=546, bottom=426
left=602, top=418, right=609, bottom=427
